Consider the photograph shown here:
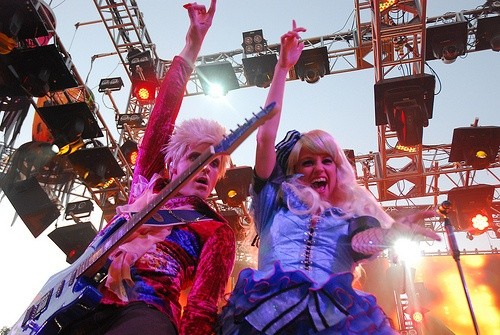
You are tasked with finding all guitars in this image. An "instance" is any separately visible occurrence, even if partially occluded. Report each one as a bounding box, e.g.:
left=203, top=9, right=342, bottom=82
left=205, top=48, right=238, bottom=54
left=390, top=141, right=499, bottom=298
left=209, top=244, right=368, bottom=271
left=0, top=102, right=281, bottom=335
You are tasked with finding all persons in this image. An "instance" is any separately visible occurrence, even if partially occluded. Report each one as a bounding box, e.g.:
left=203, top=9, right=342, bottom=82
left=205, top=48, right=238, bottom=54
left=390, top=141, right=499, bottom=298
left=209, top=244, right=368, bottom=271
left=58, top=0, right=235, bottom=335
left=217, top=18, right=442, bottom=335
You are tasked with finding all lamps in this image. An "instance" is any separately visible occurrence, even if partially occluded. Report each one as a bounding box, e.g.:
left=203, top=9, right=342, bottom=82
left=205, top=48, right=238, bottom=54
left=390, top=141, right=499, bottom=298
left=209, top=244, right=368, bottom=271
left=51, top=132, right=83, bottom=155
left=472, top=148, right=489, bottom=170
left=255, top=72, right=273, bottom=88
left=433, top=40, right=460, bottom=63
left=467, top=214, right=489, bottom=235
left=299, top=62, right=325, bottom=84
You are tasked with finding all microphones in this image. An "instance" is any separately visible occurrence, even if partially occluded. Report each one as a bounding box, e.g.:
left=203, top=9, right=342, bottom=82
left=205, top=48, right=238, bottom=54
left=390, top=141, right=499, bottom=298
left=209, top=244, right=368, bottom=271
left=435, top=201, right=452, bottom=216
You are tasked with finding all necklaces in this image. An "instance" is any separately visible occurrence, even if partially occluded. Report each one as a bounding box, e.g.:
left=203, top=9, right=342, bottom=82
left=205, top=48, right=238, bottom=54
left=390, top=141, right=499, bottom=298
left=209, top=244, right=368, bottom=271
left=162, top=205, right=208, bottom=224
